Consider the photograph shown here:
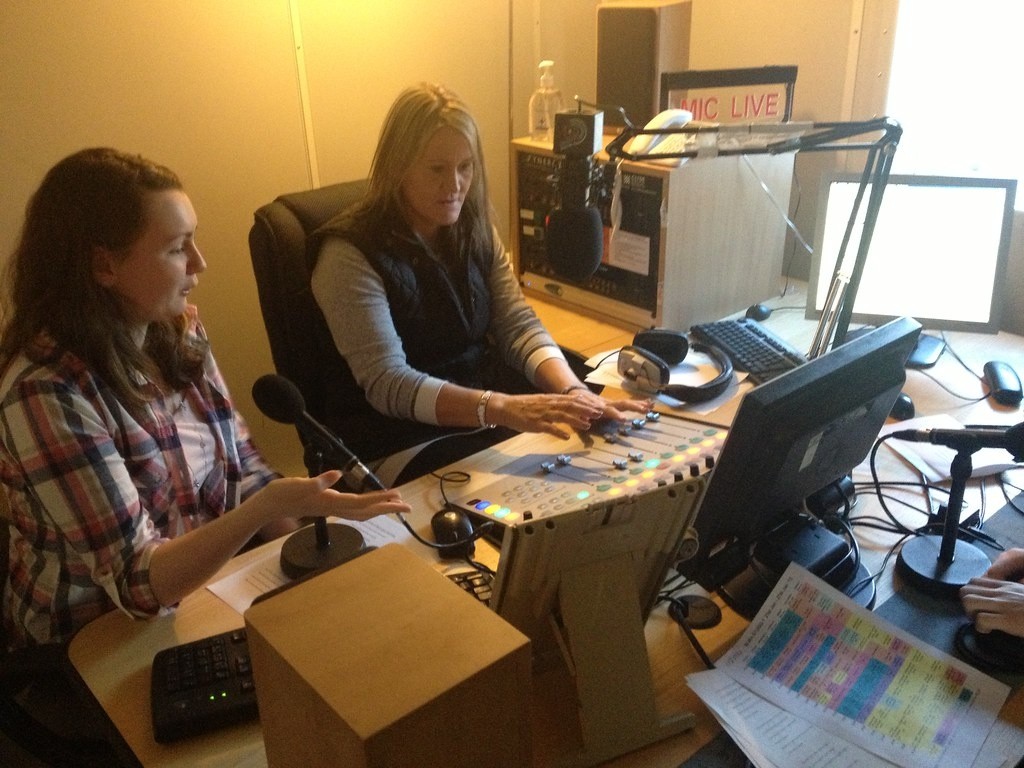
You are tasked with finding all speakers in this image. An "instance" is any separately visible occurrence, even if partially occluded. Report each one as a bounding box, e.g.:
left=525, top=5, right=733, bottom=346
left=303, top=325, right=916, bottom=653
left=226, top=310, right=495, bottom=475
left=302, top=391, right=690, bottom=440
left=595, top=0, right=692, bottom=134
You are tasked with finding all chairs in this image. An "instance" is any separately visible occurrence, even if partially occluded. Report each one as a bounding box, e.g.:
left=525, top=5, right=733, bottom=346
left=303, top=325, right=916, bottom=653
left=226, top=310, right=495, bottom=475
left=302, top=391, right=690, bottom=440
left=248, top=179, right=603, bottom=488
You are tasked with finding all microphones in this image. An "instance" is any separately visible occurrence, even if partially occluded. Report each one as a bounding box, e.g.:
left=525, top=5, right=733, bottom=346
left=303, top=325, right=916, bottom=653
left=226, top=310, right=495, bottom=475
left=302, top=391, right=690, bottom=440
left=891, top=420, right=1024, bottom=456
left=252, top=374, right=388, bottom=493
left=542, top=107, right=606, bottom=282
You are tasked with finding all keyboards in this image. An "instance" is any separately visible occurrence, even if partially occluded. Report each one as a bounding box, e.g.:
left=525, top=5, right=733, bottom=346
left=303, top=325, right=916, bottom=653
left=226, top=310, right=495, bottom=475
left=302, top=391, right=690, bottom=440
left=690, top=318, right=807, bottom=385
left=151, top=568, right=495, bottom=741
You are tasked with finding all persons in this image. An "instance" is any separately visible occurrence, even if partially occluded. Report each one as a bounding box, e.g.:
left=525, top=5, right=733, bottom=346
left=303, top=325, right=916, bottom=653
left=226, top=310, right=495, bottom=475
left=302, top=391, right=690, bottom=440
left=959, top=547, right=1024, bottom=636
left=304, top=82, right=651, bottom=489
left=1, top=146, right=412, bottom=658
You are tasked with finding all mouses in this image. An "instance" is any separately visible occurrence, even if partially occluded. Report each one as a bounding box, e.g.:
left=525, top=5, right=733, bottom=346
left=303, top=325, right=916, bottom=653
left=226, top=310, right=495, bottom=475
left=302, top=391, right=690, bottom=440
left=431, top=508, right=475, bottom=560
left=746, top=304, right=773, bottom=321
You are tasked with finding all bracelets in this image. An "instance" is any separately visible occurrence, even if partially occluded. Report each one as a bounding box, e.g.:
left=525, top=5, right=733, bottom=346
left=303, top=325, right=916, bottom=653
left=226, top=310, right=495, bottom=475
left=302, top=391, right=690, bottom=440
left=477, top=389, right=497, bottom=430
left=560, top=384, right=589, bottom=395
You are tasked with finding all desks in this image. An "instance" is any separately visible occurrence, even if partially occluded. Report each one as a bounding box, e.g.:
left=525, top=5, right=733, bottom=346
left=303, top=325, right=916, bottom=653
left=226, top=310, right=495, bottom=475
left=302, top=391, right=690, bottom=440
left=578, top=297, right=1024, bottom=516
left=519, top=275, right=810, bottom=355
left=68, top=405, right=1024, bottom=768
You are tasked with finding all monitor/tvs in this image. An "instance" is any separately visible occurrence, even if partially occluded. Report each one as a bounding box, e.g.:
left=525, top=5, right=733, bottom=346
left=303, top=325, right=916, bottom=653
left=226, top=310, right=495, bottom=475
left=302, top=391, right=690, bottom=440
left=489, top=475, right=696, bottom=768
left=803, top=169, right=1017, bottom=371
left=691, top=317, right=921, bottom=624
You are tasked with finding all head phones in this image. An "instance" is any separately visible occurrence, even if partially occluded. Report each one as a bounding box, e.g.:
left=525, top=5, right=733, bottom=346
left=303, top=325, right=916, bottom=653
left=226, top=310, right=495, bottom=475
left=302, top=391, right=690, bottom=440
left=618, top=330, right=734, bottom=404
